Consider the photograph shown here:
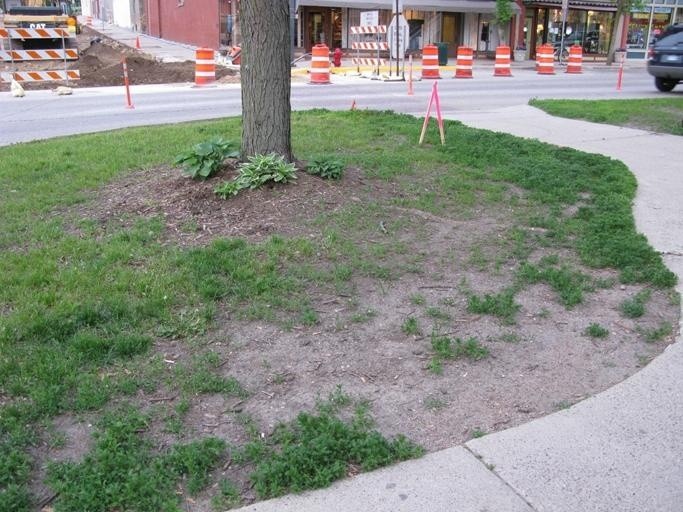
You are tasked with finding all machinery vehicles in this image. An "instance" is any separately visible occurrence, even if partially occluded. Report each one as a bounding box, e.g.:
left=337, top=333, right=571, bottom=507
left=0, top=0, right=77, bottom=49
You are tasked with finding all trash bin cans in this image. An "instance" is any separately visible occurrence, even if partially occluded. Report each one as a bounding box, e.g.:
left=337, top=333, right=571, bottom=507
left=76, top=24, right=81, bottom=34
left=614, top=49, right=627, bottom=63
left=433, top=43, right=448, bottom=66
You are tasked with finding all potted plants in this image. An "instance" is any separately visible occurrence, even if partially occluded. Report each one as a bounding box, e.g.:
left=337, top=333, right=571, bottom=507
left=513, top=46, right=527, bottom=62
left=614, top=48, right=627, bottom=63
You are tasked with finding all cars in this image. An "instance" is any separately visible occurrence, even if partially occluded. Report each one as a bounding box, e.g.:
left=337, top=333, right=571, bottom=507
left=645, top=23, right=683, bottom=91
left=557, top=30, right=608, bottom=56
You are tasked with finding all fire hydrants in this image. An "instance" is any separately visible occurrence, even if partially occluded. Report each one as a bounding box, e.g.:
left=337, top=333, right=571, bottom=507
left=331, top=48, right=344, bottom=67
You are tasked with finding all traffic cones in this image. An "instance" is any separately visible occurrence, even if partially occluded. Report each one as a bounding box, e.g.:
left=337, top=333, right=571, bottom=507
left=135, top=32, right=141, bottom=49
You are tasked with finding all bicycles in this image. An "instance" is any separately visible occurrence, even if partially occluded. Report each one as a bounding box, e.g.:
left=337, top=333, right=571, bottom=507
left=554, top=44, right=568, bottom=68
